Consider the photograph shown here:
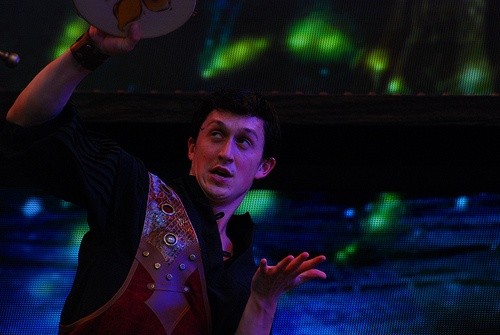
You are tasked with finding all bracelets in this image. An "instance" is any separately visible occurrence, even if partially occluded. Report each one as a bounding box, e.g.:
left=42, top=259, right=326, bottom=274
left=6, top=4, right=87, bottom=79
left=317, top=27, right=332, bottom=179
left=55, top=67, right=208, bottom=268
left=70, top=30, right=110, bottom=74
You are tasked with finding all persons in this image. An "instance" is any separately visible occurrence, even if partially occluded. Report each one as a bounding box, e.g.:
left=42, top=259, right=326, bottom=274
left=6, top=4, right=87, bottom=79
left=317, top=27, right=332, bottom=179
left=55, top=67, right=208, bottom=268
left=4, top=21, right=327, bottom=335
